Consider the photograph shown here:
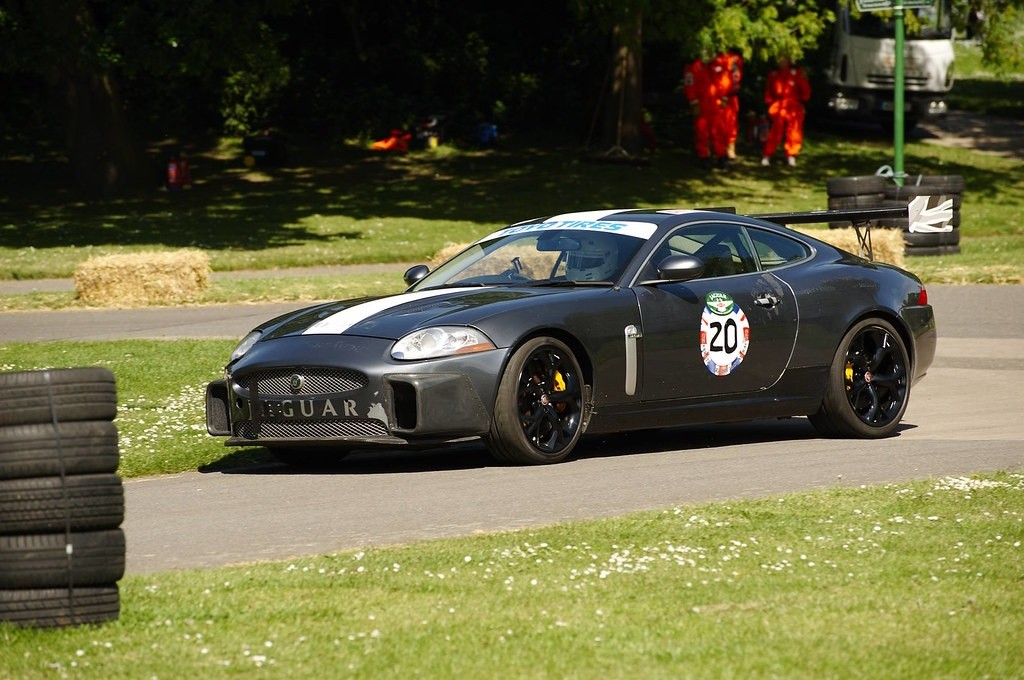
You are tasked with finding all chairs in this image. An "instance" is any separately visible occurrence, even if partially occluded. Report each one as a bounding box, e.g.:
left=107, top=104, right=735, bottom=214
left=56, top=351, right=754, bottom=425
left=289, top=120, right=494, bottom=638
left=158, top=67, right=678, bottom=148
left=618, top=238, right=672, bottom=277
left=692, top=244, right=737, bottom=279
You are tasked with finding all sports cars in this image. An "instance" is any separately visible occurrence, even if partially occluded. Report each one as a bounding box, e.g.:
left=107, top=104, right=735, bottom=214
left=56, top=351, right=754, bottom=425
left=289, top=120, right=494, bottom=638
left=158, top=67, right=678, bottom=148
left=206, top=209, right=937, bottom=464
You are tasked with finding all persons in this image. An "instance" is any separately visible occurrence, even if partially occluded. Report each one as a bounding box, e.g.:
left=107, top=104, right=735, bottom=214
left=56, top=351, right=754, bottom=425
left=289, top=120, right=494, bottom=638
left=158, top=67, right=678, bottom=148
left=564, top=232, right=618, bottom=282
left=685, top=42, right=811, bottom=167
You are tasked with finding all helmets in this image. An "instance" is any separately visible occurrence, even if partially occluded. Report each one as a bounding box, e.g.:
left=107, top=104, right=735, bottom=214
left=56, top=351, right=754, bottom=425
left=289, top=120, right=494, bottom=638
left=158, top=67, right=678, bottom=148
left=564, top=234, right=621, bottom=284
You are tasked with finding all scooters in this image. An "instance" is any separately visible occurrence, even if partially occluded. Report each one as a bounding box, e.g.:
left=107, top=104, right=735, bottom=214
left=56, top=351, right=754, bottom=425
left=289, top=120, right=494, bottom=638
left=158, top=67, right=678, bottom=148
left=244, top=137, right=285, bottom=159
left=344, top=117, right=439, bottom=192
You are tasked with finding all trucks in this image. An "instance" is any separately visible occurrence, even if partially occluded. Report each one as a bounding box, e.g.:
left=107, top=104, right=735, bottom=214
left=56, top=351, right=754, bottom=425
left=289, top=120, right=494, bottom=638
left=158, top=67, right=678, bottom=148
left=818, top=0, right=955, bottom=134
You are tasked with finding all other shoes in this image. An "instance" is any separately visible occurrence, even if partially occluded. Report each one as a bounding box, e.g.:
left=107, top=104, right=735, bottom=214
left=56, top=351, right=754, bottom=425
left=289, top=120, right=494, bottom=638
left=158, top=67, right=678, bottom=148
left=728, top=143, right=737, bottom=160
left=788, top=157, right=797, bottom=166
left=761, top=157, right=771, bottom=167
